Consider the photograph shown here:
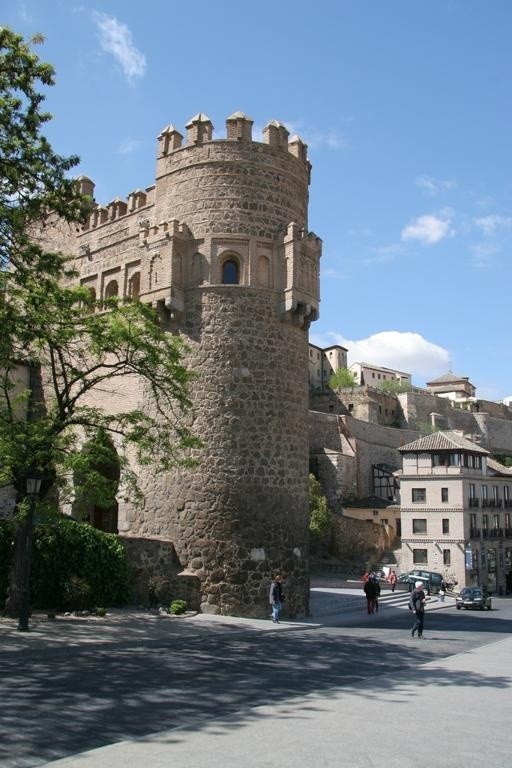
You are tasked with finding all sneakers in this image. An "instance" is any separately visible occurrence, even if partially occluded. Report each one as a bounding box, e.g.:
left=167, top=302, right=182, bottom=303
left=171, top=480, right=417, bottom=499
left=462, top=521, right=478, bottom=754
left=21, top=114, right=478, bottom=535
left=410, top=629, right=425, bottom=640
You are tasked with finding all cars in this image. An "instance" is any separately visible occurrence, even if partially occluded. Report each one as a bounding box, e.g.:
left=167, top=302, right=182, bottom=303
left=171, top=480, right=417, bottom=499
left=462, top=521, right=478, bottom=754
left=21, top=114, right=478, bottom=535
left=455, top=586, right=492, bottom=610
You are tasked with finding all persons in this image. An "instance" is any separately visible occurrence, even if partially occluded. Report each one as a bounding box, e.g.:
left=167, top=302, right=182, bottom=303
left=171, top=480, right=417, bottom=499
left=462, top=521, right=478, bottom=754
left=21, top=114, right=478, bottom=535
left=388, top=570, right=397, bottom=593
left=363, top=572, right=380, bottom=615
left=439, top=579, right=446, bottom=602
left=408, top=580, right=426, bottom=640
left=268, top=575, right=284, bottom=624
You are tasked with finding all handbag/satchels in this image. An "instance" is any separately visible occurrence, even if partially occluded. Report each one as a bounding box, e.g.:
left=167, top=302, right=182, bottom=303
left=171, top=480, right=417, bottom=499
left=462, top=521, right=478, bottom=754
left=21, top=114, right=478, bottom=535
left=408, top=600, right=421, bottom=611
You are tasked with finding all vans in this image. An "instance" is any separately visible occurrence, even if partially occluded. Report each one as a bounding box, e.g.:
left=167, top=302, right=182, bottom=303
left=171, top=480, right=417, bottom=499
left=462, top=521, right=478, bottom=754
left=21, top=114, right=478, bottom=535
left=397, top=569, right=443, bottom=594
